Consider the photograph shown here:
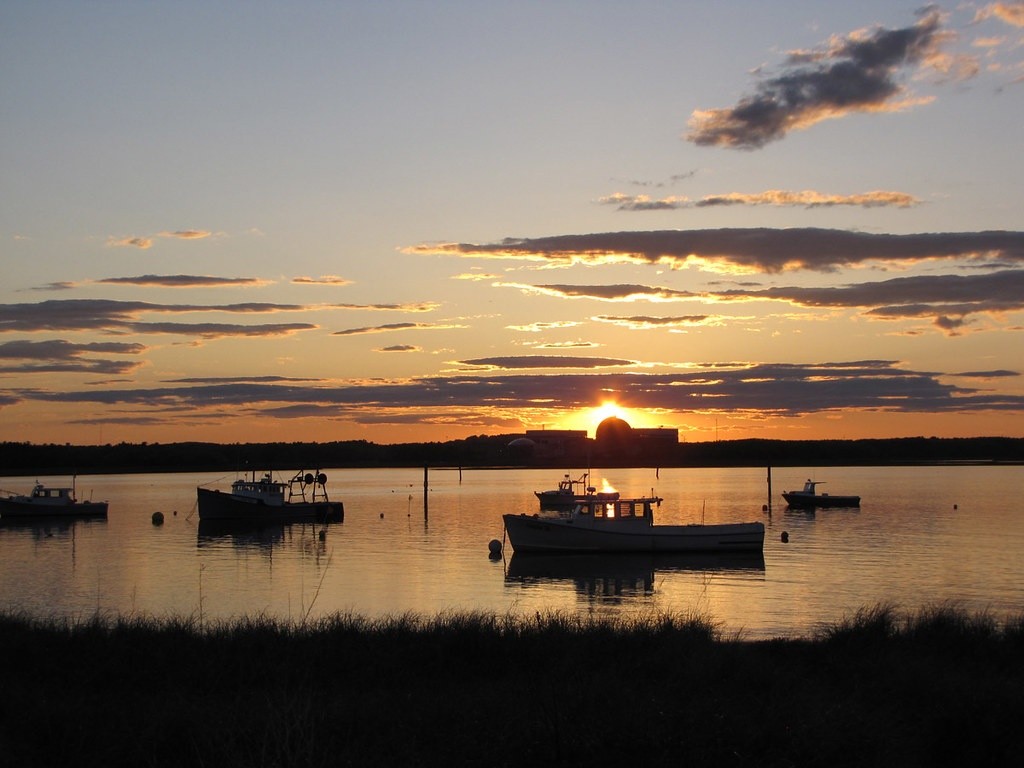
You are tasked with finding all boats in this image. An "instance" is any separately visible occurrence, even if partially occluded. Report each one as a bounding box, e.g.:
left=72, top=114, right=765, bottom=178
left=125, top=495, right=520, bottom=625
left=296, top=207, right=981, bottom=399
left=0, top=469, right=109, bottom=520
left=533, top=473, right=664, bottom=506
left=197, top=461, right=344, bottom=518
left=501, top=464, right=766, bottom=554
left=781, top=471, right=861, bottom=508
